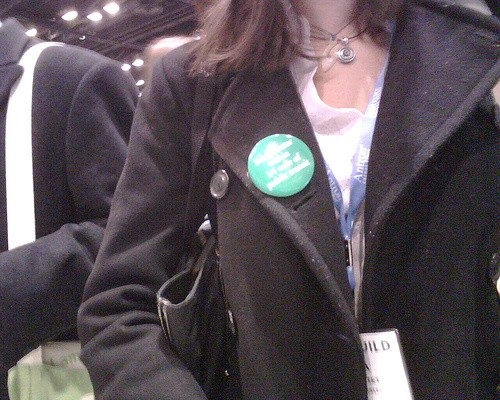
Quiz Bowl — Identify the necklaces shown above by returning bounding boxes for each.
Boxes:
[298,15,374,70]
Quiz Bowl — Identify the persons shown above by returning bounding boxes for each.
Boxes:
[0,18,139,400]
[76,0,500,400]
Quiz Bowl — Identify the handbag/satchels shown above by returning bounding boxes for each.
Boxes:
[150,235,240,400]
[6,339,96,399]
